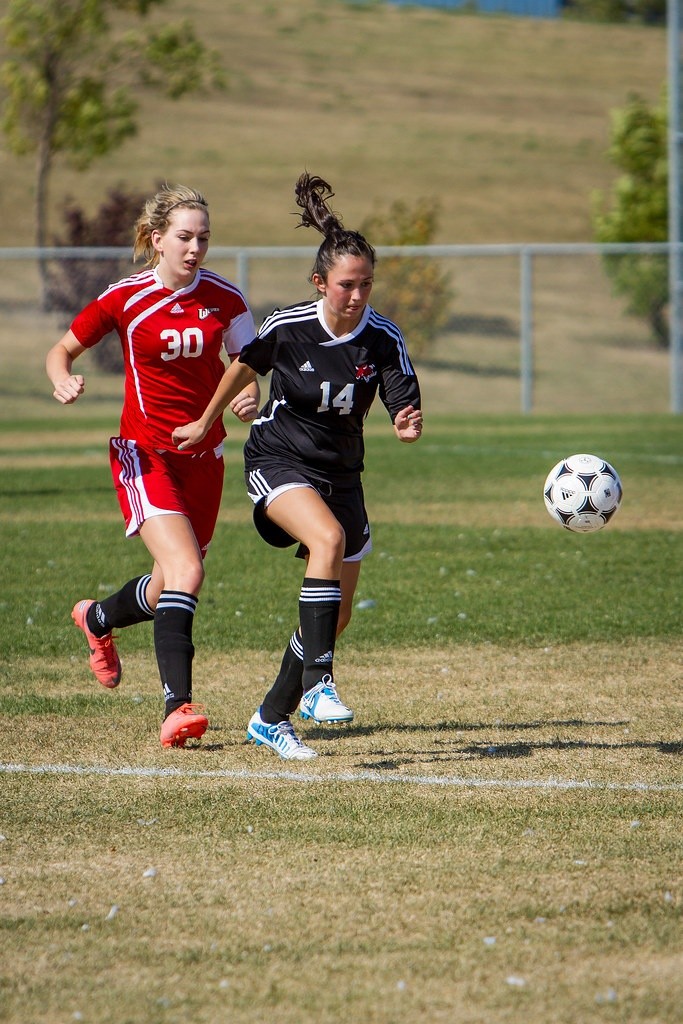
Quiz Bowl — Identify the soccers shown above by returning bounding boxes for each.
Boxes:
[543,454,623,535]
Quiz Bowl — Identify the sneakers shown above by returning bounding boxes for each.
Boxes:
[299,674,353,736]
[247,705,318,760]
[70,599,122,688]
[161,703,208,747]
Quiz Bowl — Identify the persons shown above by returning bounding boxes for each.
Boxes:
[171,173,423,760]
[46,184,259,749]
